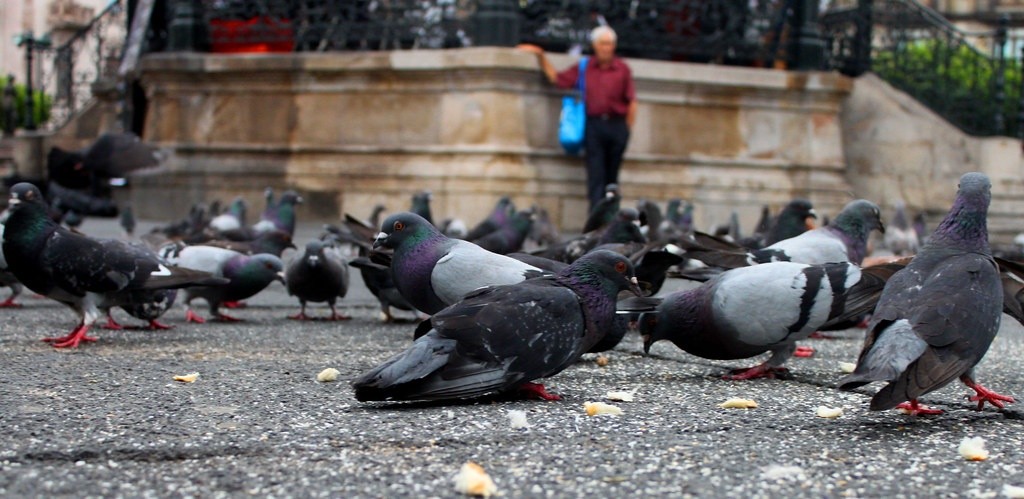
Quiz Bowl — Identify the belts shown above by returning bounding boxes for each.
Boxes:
[588,114,621,120]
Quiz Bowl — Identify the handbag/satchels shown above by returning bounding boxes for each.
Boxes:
[558,97,585,153]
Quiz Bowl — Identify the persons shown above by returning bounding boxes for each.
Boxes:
[515,26,636,213]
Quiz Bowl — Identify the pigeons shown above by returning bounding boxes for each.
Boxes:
[0,169,1015,420]
